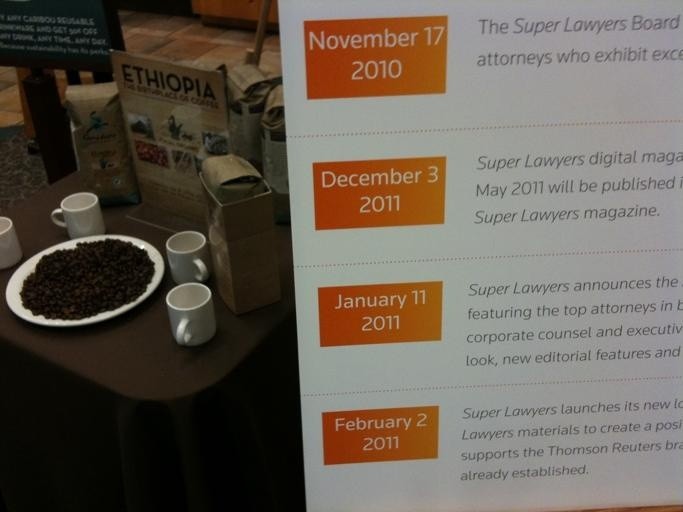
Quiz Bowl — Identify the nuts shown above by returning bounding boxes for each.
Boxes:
[19,238,155,320]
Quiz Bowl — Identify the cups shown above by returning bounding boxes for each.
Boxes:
[166,231,219,348]
[49,192,106,241]
[0,217,24,270]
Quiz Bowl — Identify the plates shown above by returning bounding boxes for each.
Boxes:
[5,235,166,329]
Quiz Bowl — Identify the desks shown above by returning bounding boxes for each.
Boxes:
[0,169,295,512]
